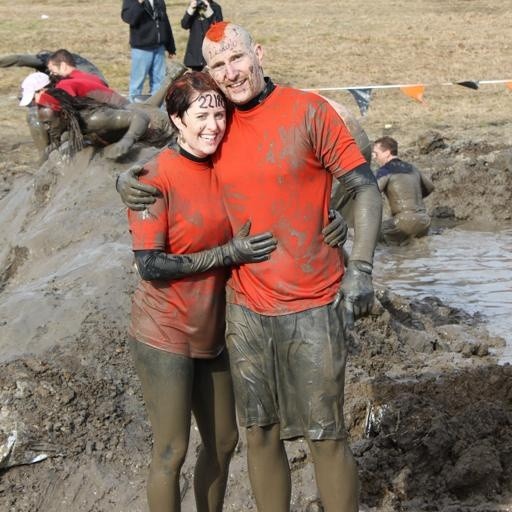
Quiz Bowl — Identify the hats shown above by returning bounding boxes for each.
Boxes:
[19,70,51,106]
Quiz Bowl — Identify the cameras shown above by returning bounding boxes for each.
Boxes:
[197,0,207,10]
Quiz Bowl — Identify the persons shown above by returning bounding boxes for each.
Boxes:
[116,22,381,511]
[121,0,177,111]
[371,136,433,243]
[126,69,349,510]
[0,48,188,161]
[182,0,223,70]
